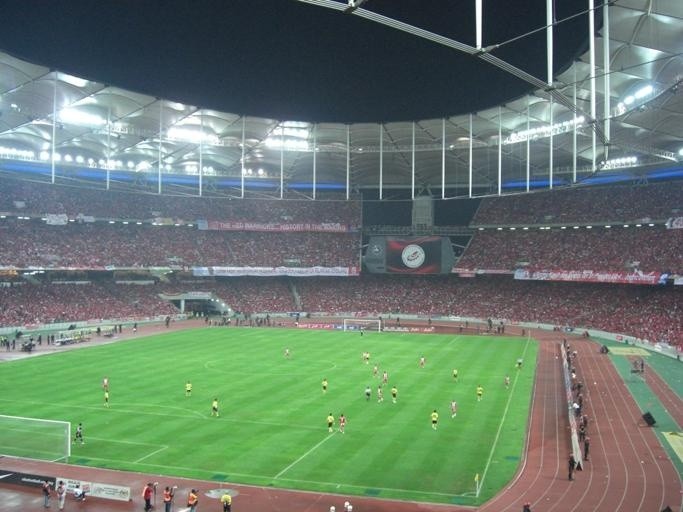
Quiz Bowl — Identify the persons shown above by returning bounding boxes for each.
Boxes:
[101,376,108,390]
[72,484,84,500]
[142,482,153,512]
[567,360,590,480]
[209,397,220,417]
[346,505,353,511]
[187,488,197,511]
[320,349,525,434]
[54,480,65,510]
[103,390,109,407]
[0,166,683,361]
[162,486,173,512]
[220,491,231,511]
[41,479,49,506]
[343,500,349,511]
[71,423,84,444]
[522,501,531,512]
[184,381,192,396]
[329,505,336,511]
